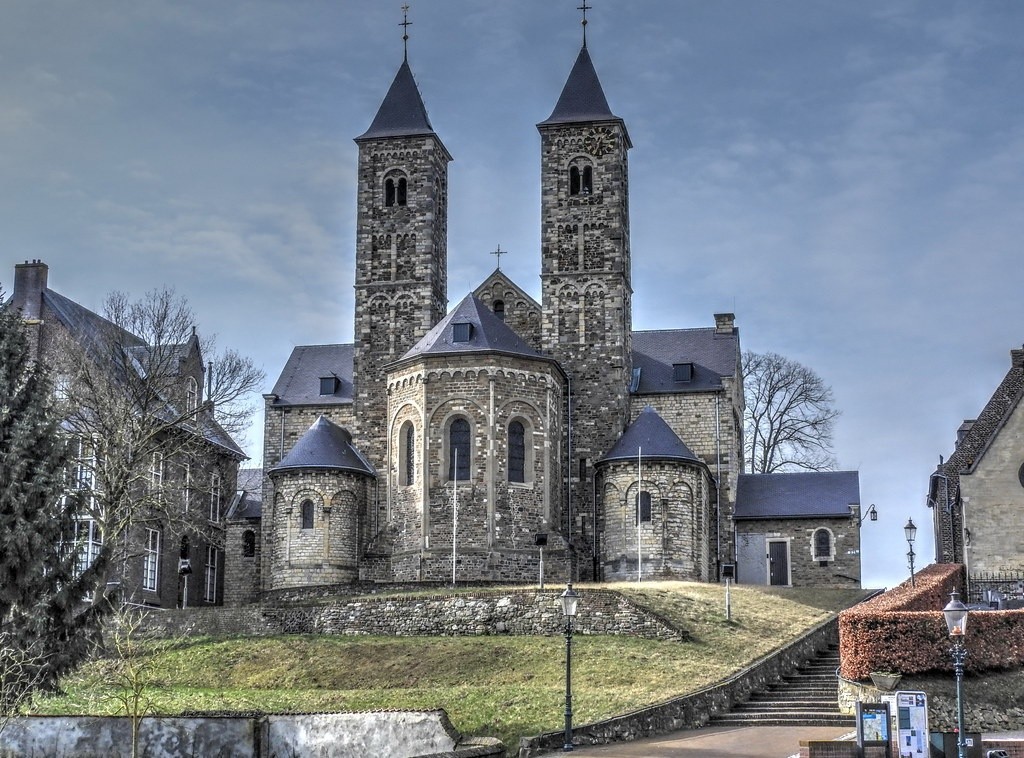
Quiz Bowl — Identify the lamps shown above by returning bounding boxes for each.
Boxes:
[179,563,194,610]
[721,564,734,622]
[860,504,877,527]
[533,533,549,588]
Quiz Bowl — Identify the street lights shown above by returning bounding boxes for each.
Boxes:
[904,517,917,585]
[559,584,580,751]
[943,586,970,758]
